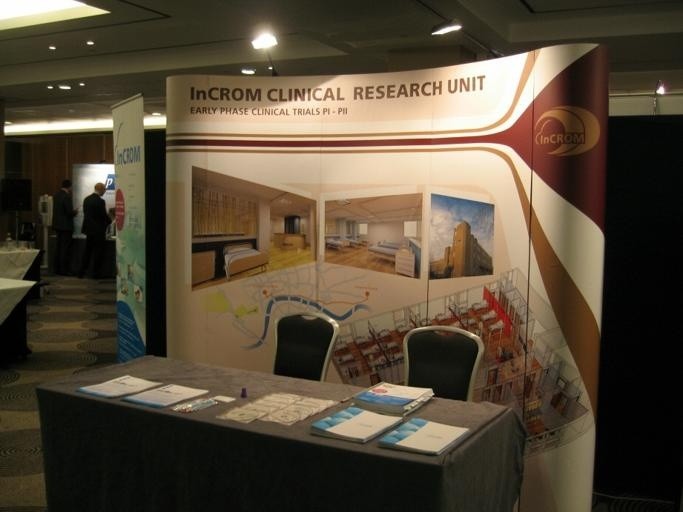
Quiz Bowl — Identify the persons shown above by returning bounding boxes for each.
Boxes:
[50,179,79,278]
[80,183,114,280]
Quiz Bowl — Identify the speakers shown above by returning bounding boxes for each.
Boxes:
[0,179,32,212]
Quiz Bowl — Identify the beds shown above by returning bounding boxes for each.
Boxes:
[218,240,272,283]
[323,230,409,264]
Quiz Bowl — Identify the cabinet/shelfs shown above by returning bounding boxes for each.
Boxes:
[0,274,49,369]
[0,243,47,302]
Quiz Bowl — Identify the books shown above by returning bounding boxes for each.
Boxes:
[309,405,404,444]
[74,374,163,400]
[120,384,209,409]
[350,380,434,417]
[375,418,471,455]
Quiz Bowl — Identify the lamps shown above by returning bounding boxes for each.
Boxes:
[427,16,464,38]
[248,27,286,78]
[650,79,666,115]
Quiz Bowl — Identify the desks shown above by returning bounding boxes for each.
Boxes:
[32,353,528,511]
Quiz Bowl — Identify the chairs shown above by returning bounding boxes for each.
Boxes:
[265,309,340,382]
[397,324,486,405]
